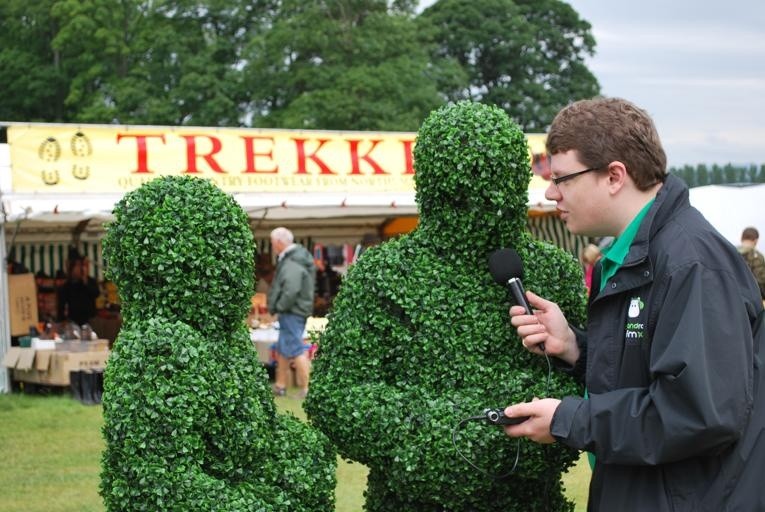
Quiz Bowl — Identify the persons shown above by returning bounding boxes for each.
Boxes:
[54,253,102,339]
[581,243,602,264]
[8,259,25,275]
[733,226,765,297]
[265,227,316,400]
[256,269,271,292]
[498,96,765,512]
[317,257,344,301]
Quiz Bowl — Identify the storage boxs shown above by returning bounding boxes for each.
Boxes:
[1,273,112,387]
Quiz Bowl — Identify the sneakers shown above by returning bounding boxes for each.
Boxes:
[273,386,287,396]
[291,389,308,399]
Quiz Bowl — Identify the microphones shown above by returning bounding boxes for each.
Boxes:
[488,248,545,351]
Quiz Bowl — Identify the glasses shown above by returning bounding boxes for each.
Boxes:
[549,166,606,189]
